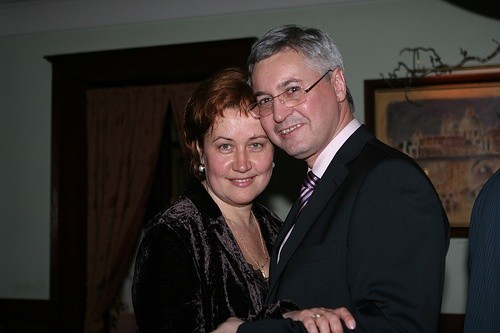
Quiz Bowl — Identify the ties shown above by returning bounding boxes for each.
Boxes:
[294,170,320,226]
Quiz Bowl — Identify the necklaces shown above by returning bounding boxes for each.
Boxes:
[222,207,269,270]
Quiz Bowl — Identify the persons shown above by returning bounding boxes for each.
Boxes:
[202,22,452,333]
[131,69,357,333]
[462,164,500,333]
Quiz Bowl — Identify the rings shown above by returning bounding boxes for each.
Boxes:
[311,313,324,322]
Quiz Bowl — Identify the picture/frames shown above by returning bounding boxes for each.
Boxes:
[364,72,500,240]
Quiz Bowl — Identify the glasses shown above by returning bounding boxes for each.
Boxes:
[248,69,334,118]
